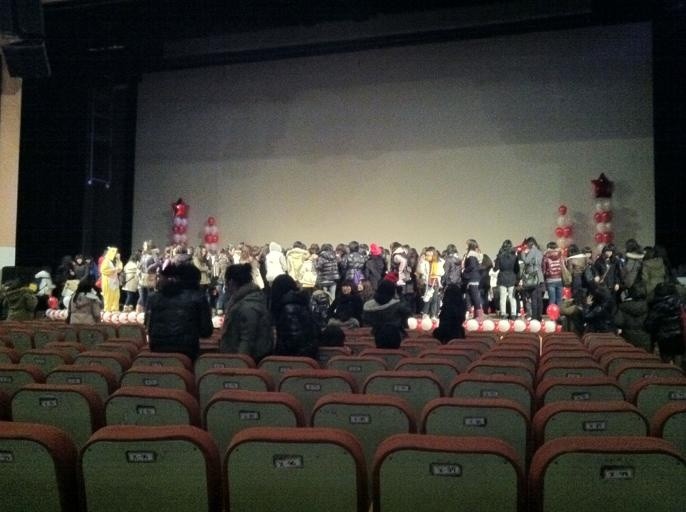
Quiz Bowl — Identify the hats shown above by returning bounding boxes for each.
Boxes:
[370,243,383,256]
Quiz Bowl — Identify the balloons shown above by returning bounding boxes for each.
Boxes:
[204,216,219,243]
[554,204,575,247]
[591,171,616,253]
[172,198,190,243]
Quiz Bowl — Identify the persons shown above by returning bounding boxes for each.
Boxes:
[3,239,686,368]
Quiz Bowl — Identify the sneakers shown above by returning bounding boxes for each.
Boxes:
[103,305,144,312]
[422,312,543,321]
